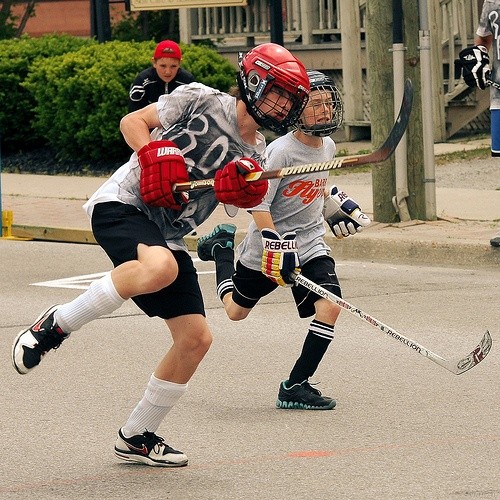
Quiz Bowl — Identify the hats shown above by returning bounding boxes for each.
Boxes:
[153,40,181,60]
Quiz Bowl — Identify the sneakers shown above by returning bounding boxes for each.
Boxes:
[113,427,188,467]
[11,304,70,374]
[275,380,336,410]
[196,224,237,261]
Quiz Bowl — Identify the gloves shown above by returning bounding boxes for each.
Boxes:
[323,185,372,239]
[459,45,494,90]
[260,228,303,287]
[214,158,268,208]
[137,139,190,211]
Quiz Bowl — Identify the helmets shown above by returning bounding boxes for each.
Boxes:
[293,70,342,137]
[237,42,310,136]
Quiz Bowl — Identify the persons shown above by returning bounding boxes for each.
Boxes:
[197,70,371,411]
[128,39,196,133]
[11,43,310,468]
[473,0,500,246]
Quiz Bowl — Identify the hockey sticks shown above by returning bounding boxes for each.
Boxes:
[485,78,500,92]
[171,77,413,193]
[296,272,493,376]
